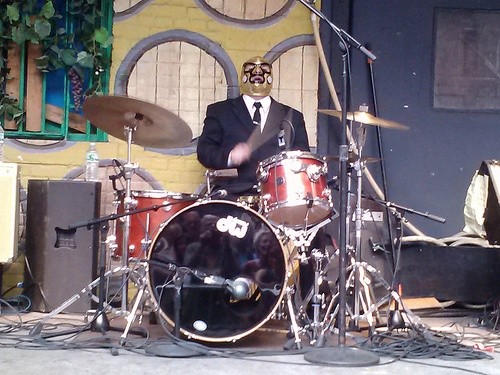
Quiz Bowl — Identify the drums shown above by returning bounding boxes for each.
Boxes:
[114,189,206,262]
[256,150,331,226]
[146,199,300,342]
[237,196,260,212]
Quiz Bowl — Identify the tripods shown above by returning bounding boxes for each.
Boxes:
[281,0,449,349]
[29,124,156,356]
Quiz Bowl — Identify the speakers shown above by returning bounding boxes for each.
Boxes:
[21,179,103,315]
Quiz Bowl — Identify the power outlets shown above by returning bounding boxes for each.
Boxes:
[362,212,384,222]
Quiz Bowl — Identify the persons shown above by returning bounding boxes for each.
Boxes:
[197,56,327,339]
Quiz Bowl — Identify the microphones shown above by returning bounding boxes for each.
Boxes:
[203,275,251,300]
[217,189,228,198]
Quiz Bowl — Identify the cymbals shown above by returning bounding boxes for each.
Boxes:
[314,109,410,131]
[329,153,380,162]
[82,96,194,149]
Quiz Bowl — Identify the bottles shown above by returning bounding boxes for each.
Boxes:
[85,143,99,181]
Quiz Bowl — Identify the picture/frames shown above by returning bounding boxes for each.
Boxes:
[429,6,500,114]
